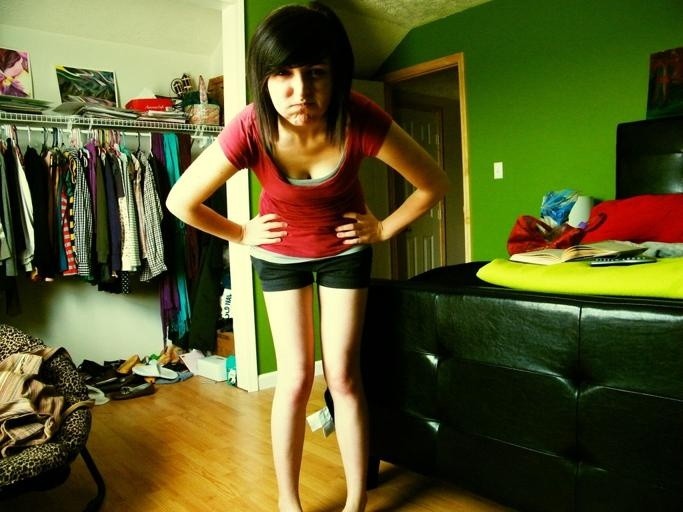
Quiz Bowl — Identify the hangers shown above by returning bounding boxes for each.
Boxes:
[0,117,146,156]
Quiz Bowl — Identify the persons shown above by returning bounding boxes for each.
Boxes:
[164,2,450,512]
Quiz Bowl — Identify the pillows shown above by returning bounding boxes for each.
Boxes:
[580,193,683,244]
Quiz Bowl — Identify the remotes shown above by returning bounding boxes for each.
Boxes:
[590,256,657,266]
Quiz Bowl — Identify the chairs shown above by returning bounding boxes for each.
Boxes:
[0,326,106,510]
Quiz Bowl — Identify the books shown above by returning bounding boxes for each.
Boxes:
[509,240,649,266]
[0,96,188,124]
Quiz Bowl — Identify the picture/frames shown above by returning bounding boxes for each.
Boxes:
[1,47,121,109]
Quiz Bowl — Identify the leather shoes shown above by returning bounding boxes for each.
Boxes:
[77,344,191,407]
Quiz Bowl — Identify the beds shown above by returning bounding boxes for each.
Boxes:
[358,118,683,510]
